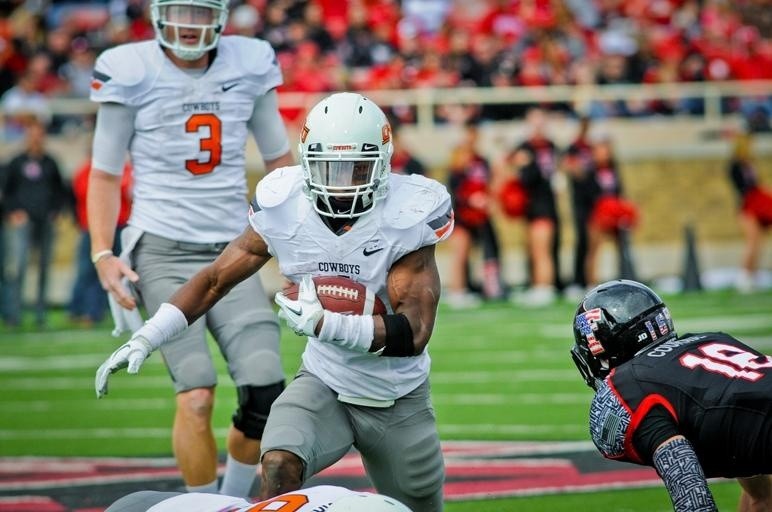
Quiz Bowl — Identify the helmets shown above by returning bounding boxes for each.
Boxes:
[568,278,676,394]
[299,91,396,219]
[150,1,231,63]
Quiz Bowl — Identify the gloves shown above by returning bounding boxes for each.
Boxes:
[274,274,326,339]
[94,333,154,399]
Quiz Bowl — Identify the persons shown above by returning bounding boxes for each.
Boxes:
[218,0,772,126]
[450,110,635,308]
[86,0,299,493]
[69,160,133,329]
[1,120,68,326]
[1,1,157,137]
[391,133,426,175]
[94,92,456,511]
[570,278,770,511]
[729,134,772,292]
[104,484,414,512]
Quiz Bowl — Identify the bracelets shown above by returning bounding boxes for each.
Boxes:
[372,309,414,358]
[317,309,375,351]
[90,249,114,262]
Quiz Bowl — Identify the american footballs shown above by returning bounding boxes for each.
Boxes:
[282,276,387,316]
